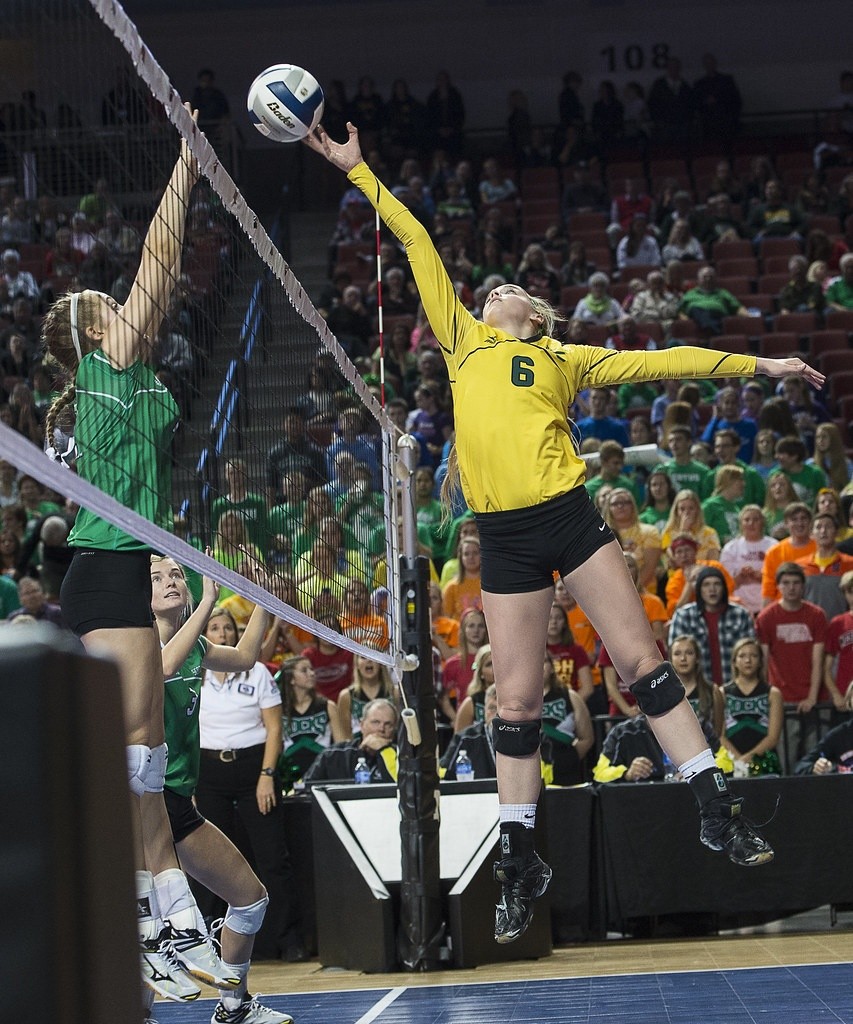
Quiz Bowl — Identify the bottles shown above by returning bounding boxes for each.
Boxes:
[354,757,370,784]
[455,750,473,781]
[663,751,677,782]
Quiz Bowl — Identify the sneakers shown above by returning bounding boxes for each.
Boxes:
[144,1007,160,1024]
[699,793,780,866]
[141,918,202,1003]
[494,849,552,944]
[210,992,295,1024]
[171,915,240,990]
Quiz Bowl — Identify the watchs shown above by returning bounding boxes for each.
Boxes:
[261,767,275,778]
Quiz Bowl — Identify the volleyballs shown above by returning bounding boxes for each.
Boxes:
[246,64,324,143]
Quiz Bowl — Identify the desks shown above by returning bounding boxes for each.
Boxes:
[213,774,853,963]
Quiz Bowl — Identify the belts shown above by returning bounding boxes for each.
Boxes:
[200,743,267,763]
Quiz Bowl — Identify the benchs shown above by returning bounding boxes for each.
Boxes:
[460,105,846,136]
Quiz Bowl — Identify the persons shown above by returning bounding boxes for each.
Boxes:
[303,117,829,942]
[37,101,298,1024]
[0,69,208,621]
[194,52,852,785]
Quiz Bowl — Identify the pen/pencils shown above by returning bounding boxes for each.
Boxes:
[820,751,824,757]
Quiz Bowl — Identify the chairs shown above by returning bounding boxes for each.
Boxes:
[18,218,224,304]
[338,156,853,456]
